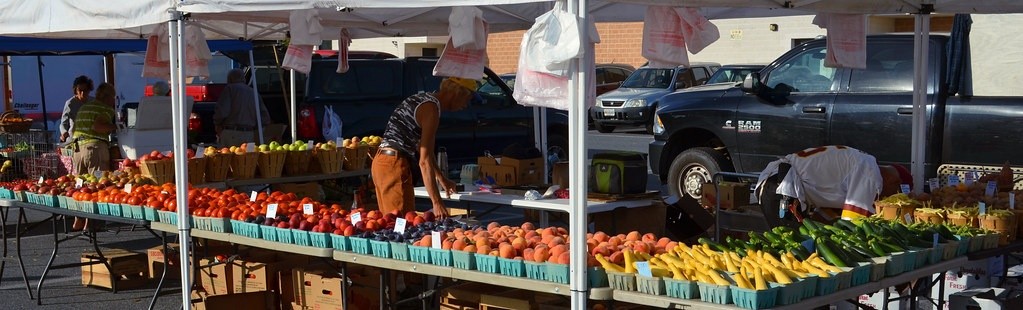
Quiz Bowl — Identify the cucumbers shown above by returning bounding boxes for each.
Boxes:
[696,216,959,266]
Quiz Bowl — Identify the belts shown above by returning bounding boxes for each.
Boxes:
[378,150,405,156]
[224,126,254,131]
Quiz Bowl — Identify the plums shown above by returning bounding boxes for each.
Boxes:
[246,206,435,236]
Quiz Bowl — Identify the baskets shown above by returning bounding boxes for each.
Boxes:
[1,143,1022,304]
[0,111,33,134]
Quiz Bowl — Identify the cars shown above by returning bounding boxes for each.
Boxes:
[477,63,637,99]
[703,63,830,93]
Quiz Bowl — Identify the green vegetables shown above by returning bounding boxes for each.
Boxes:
[12,141,30,151]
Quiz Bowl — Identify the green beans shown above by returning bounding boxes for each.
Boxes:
[876,190,1016,219]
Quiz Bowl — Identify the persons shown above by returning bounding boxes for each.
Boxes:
[59,75,94,141]
[214,69,270,147]
[371,77,477,222]
[755,144,899,232]
[71,82,116,229]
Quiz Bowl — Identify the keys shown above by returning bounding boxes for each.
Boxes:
[779,198,790,212]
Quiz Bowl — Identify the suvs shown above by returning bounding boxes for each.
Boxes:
[589,61,728,134]
[237,51,568,188]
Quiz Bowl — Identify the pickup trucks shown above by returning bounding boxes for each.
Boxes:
[646,32,1022,218]
[143,51,289,145]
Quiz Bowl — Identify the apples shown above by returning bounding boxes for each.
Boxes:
[13,168,152,197]
[123,135,383,166]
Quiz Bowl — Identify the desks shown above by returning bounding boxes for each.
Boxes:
[0,167,1023,310]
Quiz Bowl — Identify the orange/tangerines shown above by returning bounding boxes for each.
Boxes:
[72,182,341,221]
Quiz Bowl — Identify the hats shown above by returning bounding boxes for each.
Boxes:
[891,163,913,192]
[449,77,477,92]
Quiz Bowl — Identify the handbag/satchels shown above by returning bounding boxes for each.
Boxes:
[321,106,342,141]
[590,150,648,196]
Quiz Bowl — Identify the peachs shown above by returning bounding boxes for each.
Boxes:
[413,222,678,267]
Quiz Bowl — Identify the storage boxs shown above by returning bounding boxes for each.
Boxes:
[440,282,571,310]
[552,159,593,189]
[276,262,380,310]
[187,243,307,310]
[477,153,544,186]
[701,182,750,208]
[148,243,181,279]
[931,255,1004,298]
[115,95,195,160]
[145,82,228,101]
[81,250,148,292]
[590,150,650,197]
